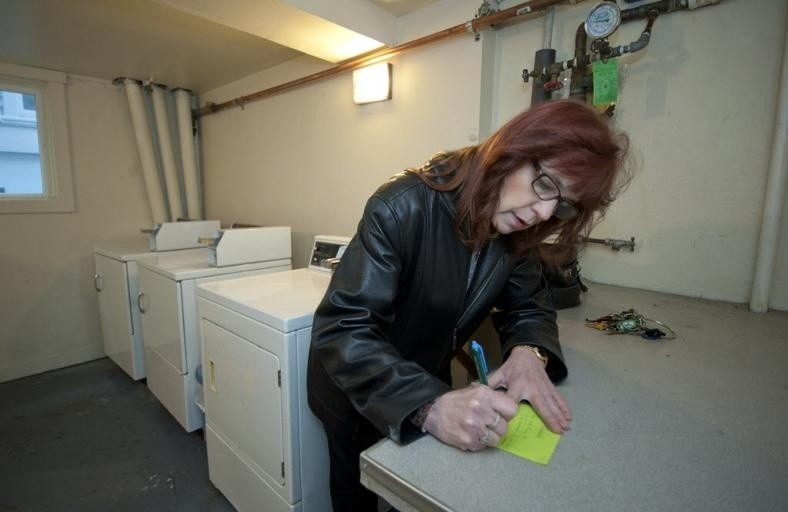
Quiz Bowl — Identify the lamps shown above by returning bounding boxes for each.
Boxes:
[351,62,391,104]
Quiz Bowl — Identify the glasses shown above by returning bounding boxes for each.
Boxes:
[531,158,580,221]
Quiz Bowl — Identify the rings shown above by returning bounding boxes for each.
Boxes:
[478,428,489,447]
[487,410,501,430]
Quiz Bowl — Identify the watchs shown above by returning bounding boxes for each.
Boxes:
[513,345,548,366]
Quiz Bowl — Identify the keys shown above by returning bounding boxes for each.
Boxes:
[583,308,647,336]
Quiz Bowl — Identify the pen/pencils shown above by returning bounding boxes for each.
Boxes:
[471,341,488,386]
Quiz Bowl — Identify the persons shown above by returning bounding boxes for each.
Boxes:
[306,95,641,512]
